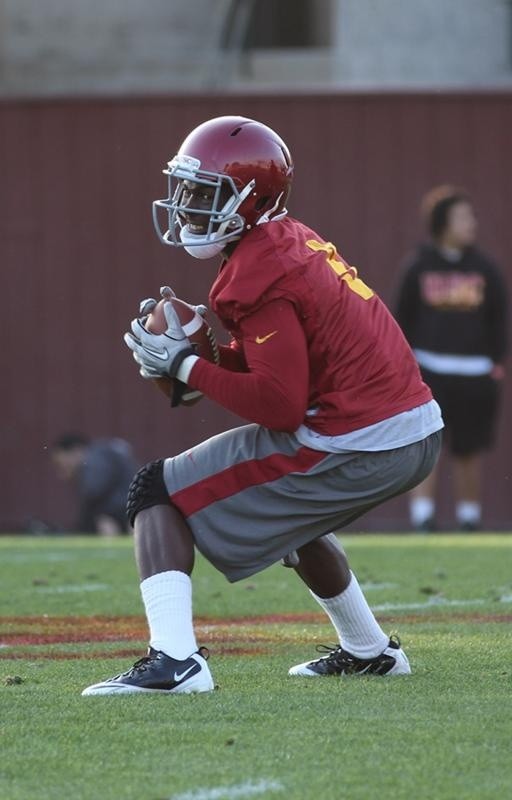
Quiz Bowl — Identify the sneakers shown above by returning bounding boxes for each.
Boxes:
[287,635,412,676]
[81,646,215,696]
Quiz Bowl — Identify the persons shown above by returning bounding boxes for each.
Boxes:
[22,418,164,538]
[389,194,510,534]
[75,111,450,699]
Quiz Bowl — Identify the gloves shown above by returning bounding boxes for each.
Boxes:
[123,285,208,382]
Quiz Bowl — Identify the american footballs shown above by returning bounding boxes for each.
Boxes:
[145,295,220,407]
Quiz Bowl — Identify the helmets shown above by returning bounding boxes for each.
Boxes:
[171,115,294,234]
